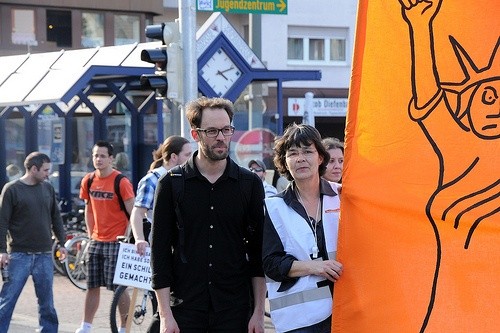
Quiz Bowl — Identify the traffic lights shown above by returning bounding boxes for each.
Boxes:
[139,22,183,103]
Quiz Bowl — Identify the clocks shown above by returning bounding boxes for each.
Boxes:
[198,47,243,98]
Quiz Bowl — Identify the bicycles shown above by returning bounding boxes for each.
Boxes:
[52,226,157,333]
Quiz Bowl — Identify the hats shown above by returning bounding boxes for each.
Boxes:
[248,160,266,172]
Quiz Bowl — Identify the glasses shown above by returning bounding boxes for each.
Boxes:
[249,167,263,172]
[195,126,235,138]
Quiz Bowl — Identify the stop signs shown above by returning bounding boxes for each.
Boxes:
[234,128,276,173]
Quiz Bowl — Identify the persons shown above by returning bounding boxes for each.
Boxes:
[147,97,267,333]
[130,136,192,314]
[75,141,135,333]
[248,158,279,198]
[6,163,22,181]
[260,121,343,333]
[0,151,68,333]
[319,137,345,184]
